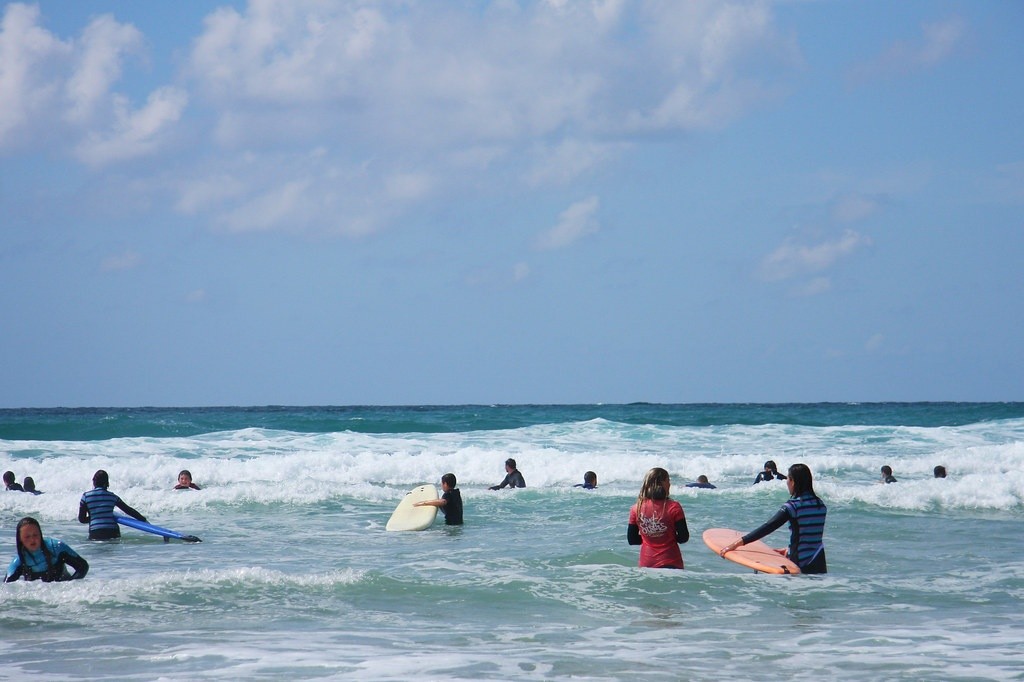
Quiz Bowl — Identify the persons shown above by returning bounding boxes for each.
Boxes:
[627,468,689,569]
[413,473,463,525]
[488,459,526,490]
[78,470,150,541]
[3,471,42,495]
[174,470,201,491]
[686,475,717,489]
[4,517,89,582]
[574,471,599,490]
[720,460,828,574]
[881,466,897,483]
[934,466,946,478]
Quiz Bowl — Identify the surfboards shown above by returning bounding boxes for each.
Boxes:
[385,485,440,531]
[114,511,201,542]
[701,527,801,575]
